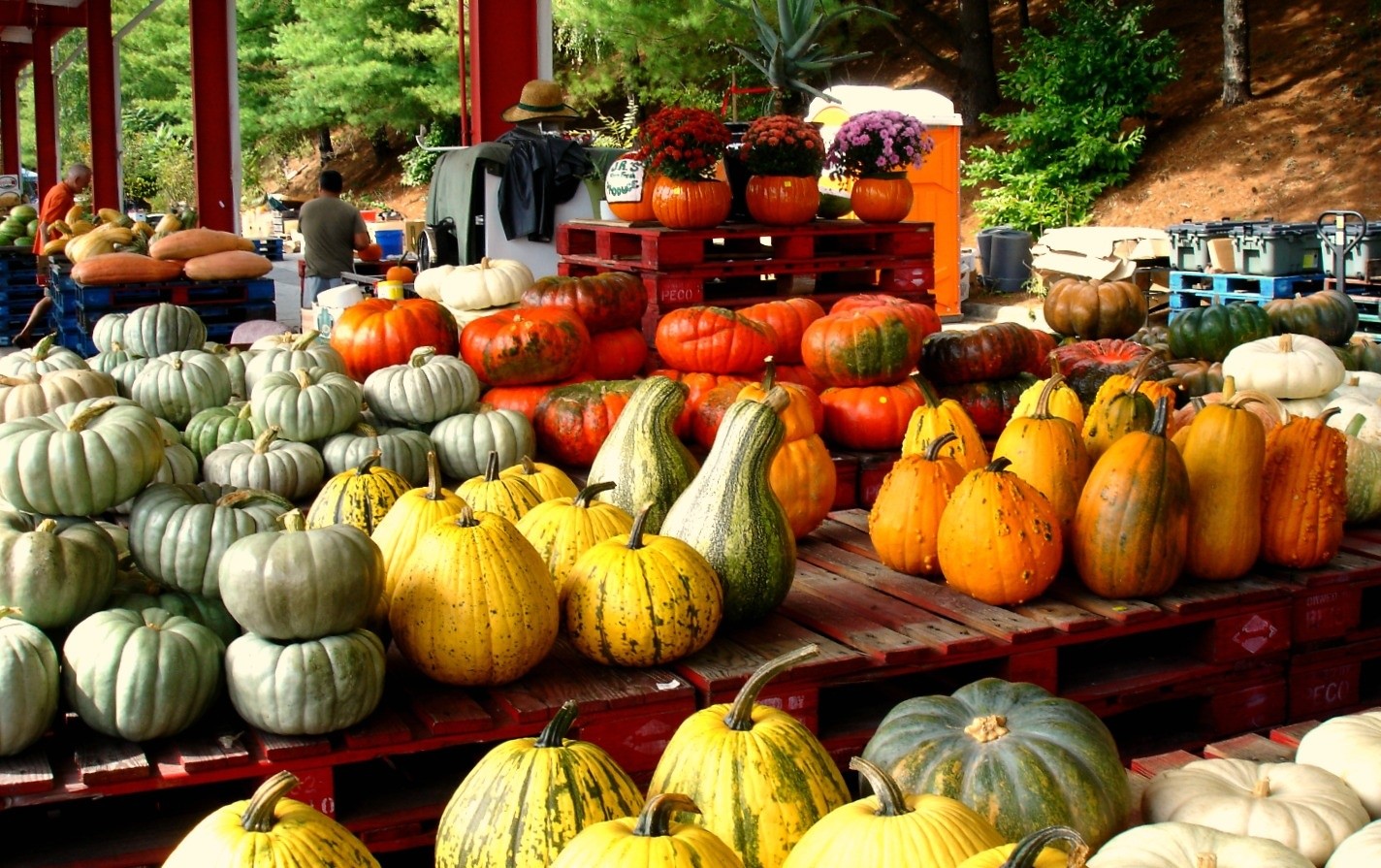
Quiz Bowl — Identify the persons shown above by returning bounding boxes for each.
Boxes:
[297,171,369,308]
[13,164,91,347]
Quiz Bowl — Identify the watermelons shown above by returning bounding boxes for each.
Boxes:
[0,205,40,247]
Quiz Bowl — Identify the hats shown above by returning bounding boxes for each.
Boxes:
[501,77,582,122]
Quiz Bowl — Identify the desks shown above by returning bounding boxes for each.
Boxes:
[551,218,936,334]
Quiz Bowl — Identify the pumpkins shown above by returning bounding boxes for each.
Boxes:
[604,150,670,222]
[824,280,1380,605]
[42,205,274,286]
[652,175,914,230]
[160,644,1381,868]
[0,258,943,764]
[358,242,383,261]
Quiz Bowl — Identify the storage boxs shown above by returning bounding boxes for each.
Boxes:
[960,262,969,302]
[961,246,976,283]
[0,256,276,360]
[1166,215,1274,272]
[1227,221,1335,275]
[1316,220,1381,278]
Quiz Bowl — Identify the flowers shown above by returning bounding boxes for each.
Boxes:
[633,107,732,184]
[740,111,828,182]
[823,109,935,191]
